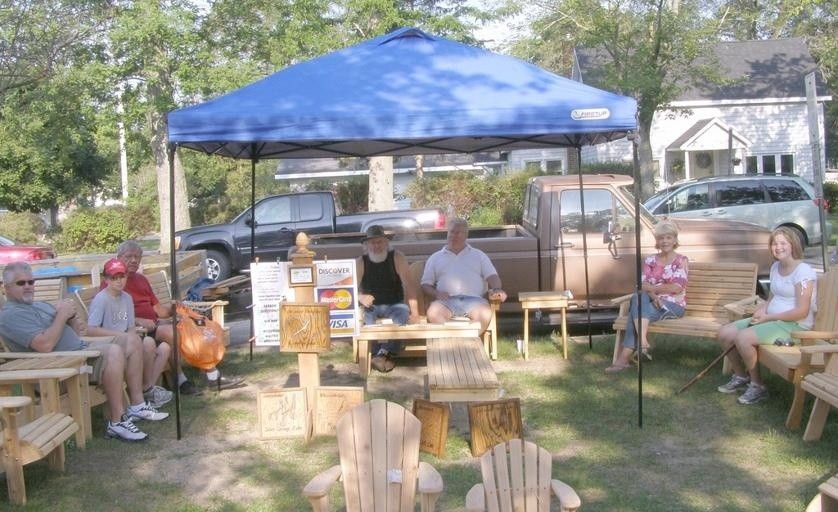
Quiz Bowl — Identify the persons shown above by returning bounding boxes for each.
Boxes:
[99,241,220,396]
[604,220,689,373]
[354,225,419,373]
[86,259,174,409]
[717,227,817,405]
[420,219,508,337]
[0,262,169,440]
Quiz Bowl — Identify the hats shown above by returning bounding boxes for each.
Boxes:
[360,226,395,243]
[103,259,127,276]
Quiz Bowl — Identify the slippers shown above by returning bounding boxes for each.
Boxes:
[633,347,652,363]
[604,363,633,374]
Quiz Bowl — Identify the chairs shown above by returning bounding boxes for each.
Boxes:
[803,471,838,511]
[0,296,134,438]
[0,367,79,507]
[465,439,582,512]
[302,399,443,512]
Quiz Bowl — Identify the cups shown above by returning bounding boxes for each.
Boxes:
[517,340,524,354]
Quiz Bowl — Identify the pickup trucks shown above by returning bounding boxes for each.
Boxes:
[175,191,446,302]
[287,173,777,314]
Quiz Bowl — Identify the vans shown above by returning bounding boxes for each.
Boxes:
[643,176,828,249]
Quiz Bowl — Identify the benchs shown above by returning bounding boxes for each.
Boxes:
[74,268,232,392]
[756,264,837,442]
[610,262,762,376]
[350,261,501,360]
[0,276,69,302]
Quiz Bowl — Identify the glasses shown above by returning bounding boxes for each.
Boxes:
[5,280,34,285]
[776,338,795,347]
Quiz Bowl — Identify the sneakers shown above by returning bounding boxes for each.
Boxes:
[738,381,770,404]
[104,385,173,441]
[718,374,750,393]
[371,353,400,372]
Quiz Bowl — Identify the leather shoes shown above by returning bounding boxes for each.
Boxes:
[180,380,202,395]
[207,377,245,391]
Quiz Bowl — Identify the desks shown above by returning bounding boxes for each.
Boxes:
[519,291,568,361]
[426,337,500,403]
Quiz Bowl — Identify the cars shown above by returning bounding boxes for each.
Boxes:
[1,235,56,295]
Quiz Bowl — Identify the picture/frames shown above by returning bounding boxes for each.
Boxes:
[467,398,524,458]
[412,398,449,457]
[310,384,364,436]
[287,264,317,287]
[278,302,330,353]
[256,387,307,440]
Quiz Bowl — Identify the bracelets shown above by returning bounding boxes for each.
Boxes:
[493,288,501,291]
